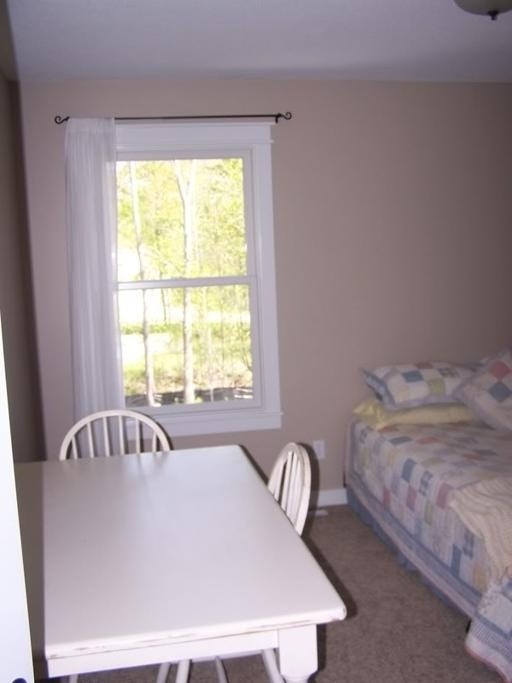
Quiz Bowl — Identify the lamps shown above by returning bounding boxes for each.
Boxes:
[455,0,512,21]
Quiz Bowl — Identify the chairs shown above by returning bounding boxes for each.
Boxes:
[57,408,172,683]
[157,442,314,683]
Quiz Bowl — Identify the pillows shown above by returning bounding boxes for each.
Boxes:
[455,346,512,432]
[355,396,476,430]
[362,357,474,412]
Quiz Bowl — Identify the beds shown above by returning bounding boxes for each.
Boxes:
[346,417,512,683]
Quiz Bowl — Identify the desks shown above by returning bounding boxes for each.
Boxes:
[16,444,348,682]
